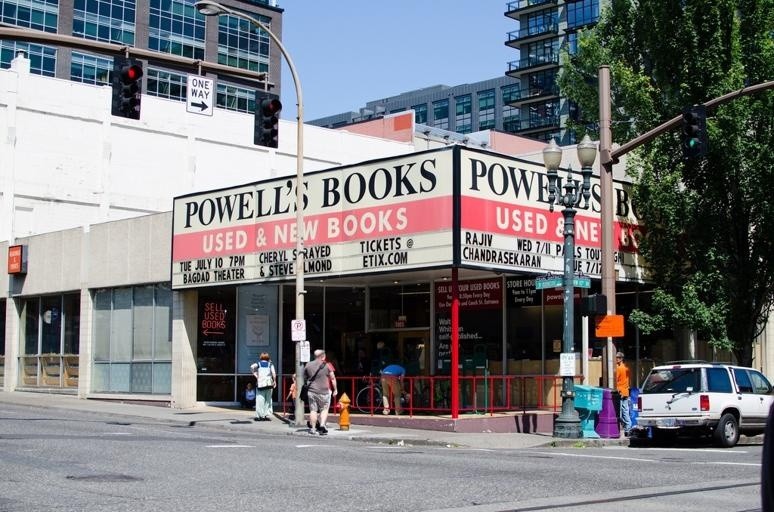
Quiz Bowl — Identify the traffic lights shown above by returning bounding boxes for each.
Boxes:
[252,89,282,149]
[681,104,709,159]
[111,54,145,121]
[583,294,609,317]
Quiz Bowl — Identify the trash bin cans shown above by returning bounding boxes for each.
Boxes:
[628,386,639,425]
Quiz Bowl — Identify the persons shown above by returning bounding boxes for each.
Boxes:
[286,373,298,421]
[250,351,278,422]
[304,349,330,436]
[382,365,407,416]
[615,351,635,434]
[245,382,256,410]
[307,351,339,428]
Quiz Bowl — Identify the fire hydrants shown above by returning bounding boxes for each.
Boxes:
[336,392,352,430]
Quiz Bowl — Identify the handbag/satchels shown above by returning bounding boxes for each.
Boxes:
[300,383,309,401]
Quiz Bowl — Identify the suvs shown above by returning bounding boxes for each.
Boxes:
[633,359,774,449]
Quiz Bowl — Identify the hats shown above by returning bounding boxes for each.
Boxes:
[616,352,625,358]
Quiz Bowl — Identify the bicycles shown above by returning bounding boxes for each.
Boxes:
[356,379,416,415]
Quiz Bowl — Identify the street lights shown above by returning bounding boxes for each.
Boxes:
[542,130,597,438]
[190,1,308,432]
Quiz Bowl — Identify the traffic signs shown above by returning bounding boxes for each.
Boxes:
[573,277,592,289]
[185,74,216,117]
[536,278,564,291]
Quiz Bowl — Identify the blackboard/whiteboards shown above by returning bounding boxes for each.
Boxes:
[506,276,544,306]
[432,307,503,370]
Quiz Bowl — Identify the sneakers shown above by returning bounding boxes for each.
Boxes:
[260,416,271,421]
[309,424,328,434]
